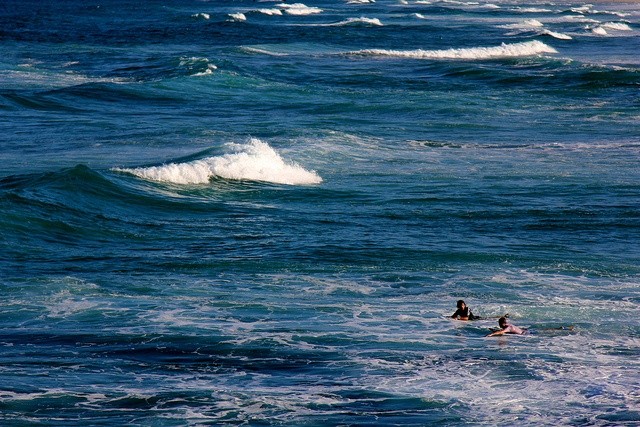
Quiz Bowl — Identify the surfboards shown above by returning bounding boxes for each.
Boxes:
[488,327,538,338]
[439,314,511,323]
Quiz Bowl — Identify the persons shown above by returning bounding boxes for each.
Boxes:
[486,314,538,338]
[452,300,482,321]
